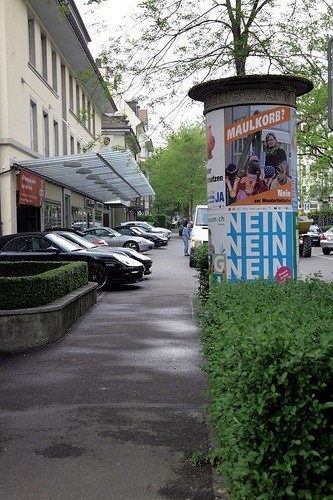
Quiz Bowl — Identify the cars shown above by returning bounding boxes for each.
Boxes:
[1,220,173,292]
[298,222,333,258]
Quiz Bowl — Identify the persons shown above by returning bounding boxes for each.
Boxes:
[225,156,291,205]
[264,133,287,180]
[182,223,192,256]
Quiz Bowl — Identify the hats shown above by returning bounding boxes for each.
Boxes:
[226,163,238,177]
[264,165,275,178]
[249,155,259,162]
[249,164,261,177]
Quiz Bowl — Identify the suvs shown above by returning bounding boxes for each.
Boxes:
[188,204,210,268]
